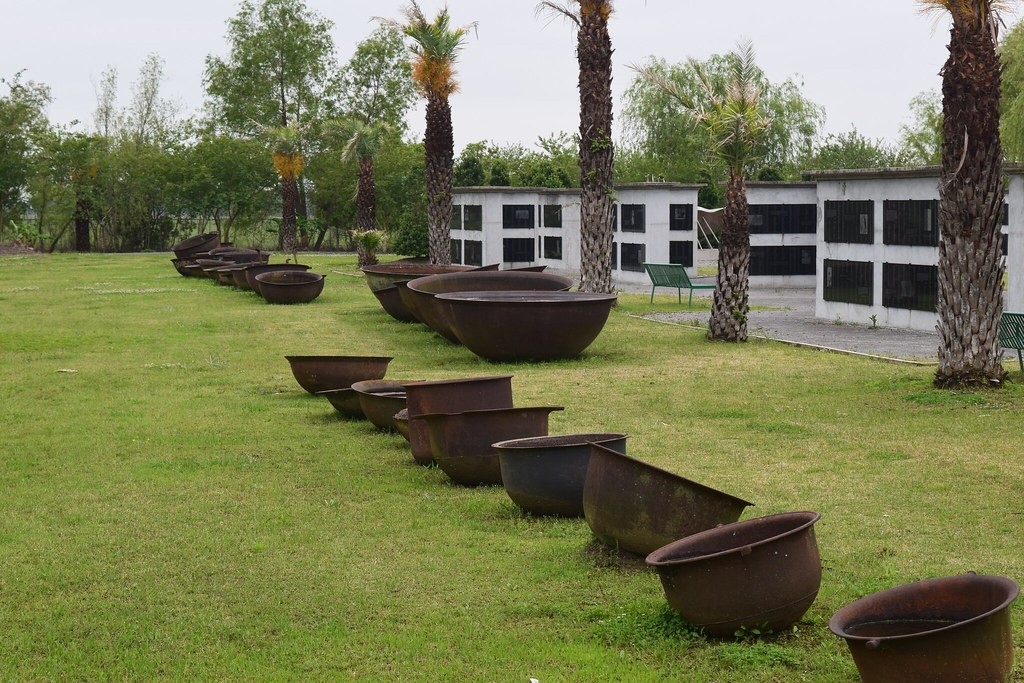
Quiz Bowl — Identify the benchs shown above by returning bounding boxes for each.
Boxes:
[643,261,715,305]
[999,313,1024,371]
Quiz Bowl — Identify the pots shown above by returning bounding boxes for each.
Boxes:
[316,388,367,419]
[491,433,631,517]
[351,379,427,430]
[435,290,618,363]
[284,356,394,397]
[360,263,483,300]
[646,511,822,640]
[401,374,514,467]
[170,231,327,305]
[583,440,756,557]
[407,271,574,346]
[393,265,548,329]
[374,263,500,323]
[393,408,411,442]
[830,571,1020,683]
[411,406,564,486]
